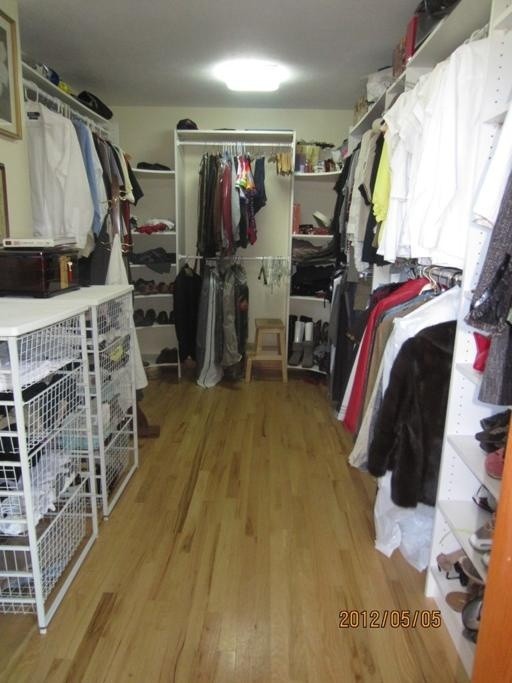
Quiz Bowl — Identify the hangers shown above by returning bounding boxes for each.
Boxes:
[23,81,109,142]
[470,27,482,41]
[259,256,287,293]
[185,253,244,268]
[461,35,470,47]
[200,140,292,158]
[399,264,462,297]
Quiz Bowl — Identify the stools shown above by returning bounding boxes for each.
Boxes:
[254,318,286,355]
[245,344,288,385]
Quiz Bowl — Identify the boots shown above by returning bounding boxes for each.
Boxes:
[288,315,330,371]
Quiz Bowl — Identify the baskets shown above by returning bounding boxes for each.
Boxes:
[1,294,131,616]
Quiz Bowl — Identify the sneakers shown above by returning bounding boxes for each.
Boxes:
[156,347,177,363]
[134,309,175,326]
[129,279,175,295]
[468,512,496,554]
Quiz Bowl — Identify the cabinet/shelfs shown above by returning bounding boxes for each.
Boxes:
[0,285,138,637]
[123,168,179,381]
[174,129,296,381]
[289,171,344,376]
[347,0,512,682]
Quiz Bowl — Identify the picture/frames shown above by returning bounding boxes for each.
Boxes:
[0,163,10,244]
[0,9,23,140]
